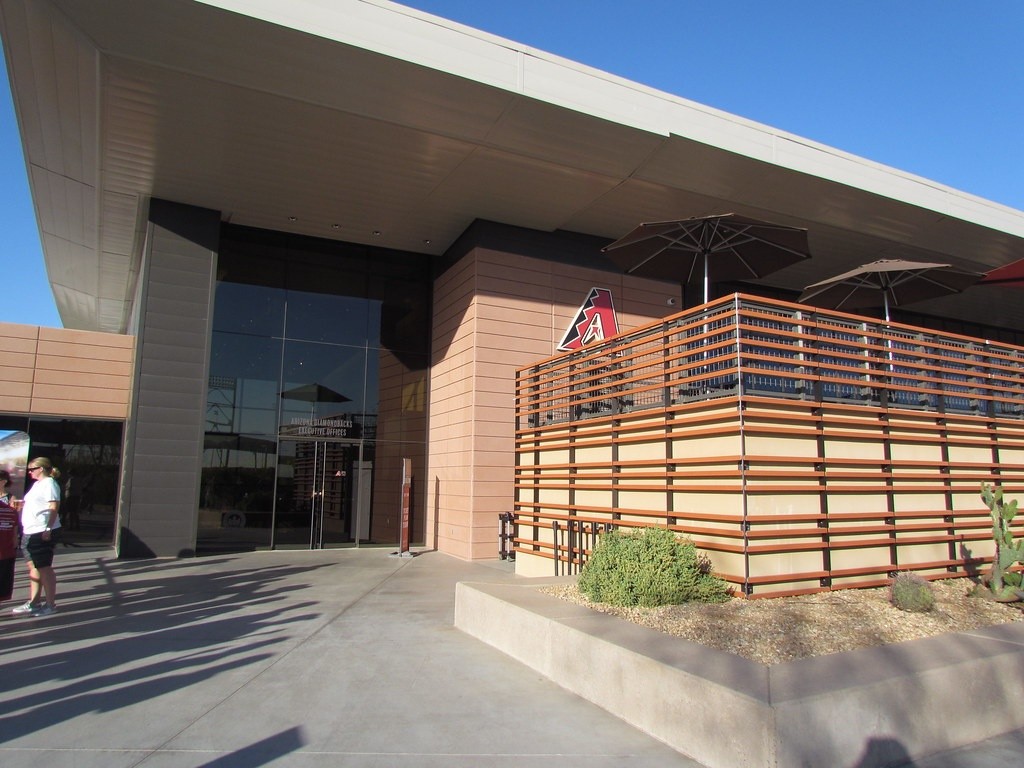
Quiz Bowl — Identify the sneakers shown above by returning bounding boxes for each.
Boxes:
[30,602,58,616]
[13,600,43,613]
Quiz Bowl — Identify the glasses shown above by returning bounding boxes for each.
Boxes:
[27,467,41,473]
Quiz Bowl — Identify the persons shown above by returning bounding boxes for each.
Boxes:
[0,470,18,509]
[13,457,62,617]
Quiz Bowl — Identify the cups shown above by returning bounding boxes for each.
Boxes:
[13,500,22,508]
